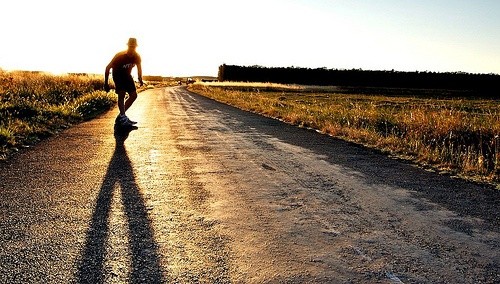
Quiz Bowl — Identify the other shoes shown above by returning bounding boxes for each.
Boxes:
[115,114,131,128]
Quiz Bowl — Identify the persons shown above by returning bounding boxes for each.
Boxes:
[104,38,143,125]
